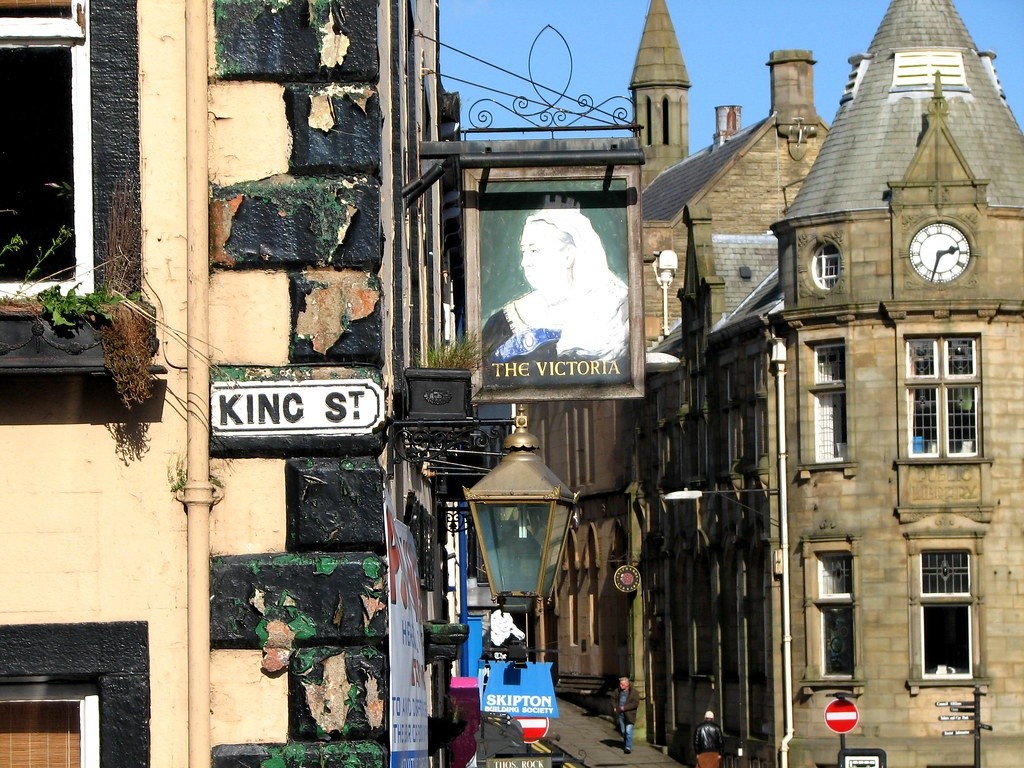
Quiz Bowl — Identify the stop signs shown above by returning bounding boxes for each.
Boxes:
[825,700,857,735]
[511,715,549,745]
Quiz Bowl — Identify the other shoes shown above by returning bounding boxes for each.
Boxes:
[621,740,633,753]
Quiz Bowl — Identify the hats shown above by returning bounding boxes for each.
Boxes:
[705,711,714,720]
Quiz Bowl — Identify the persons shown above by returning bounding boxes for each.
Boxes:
[611,677,640,754]
[695,711,724,768]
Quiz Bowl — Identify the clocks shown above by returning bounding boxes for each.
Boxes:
[911,223,971,284]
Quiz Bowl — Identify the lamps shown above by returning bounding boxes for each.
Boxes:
[386,405,580,610]
[664,488,781,524]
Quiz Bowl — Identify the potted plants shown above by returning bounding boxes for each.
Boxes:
[0,175,242,410]
